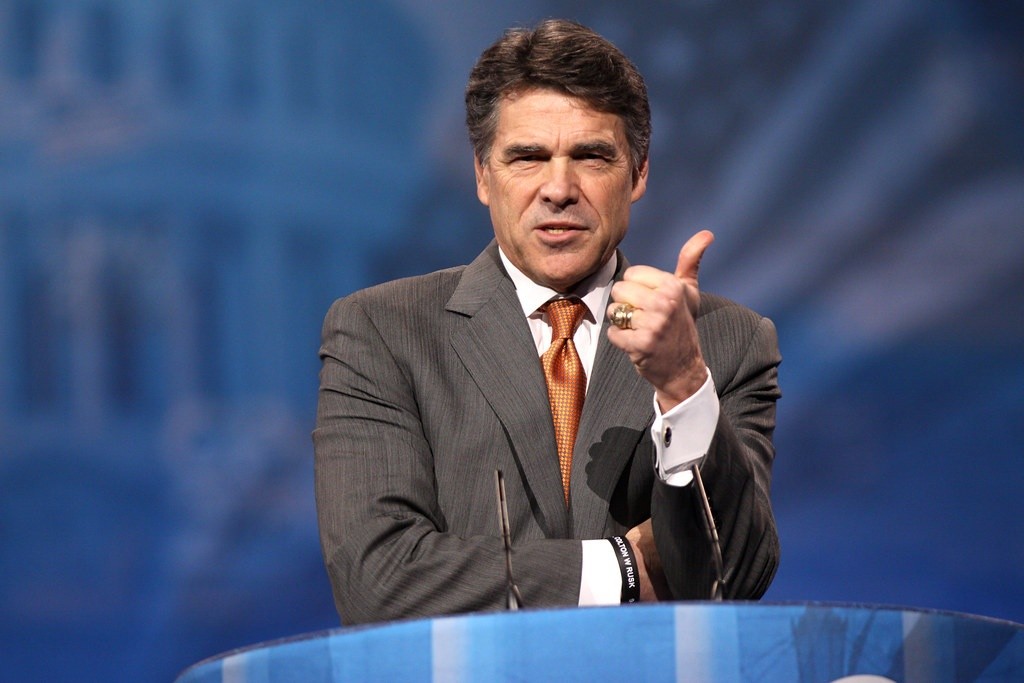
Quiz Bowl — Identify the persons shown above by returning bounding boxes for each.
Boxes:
[315,17,783,627]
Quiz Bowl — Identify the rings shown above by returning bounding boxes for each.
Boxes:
[611,300,638,331]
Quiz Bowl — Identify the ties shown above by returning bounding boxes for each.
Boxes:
[539,299,588,510]
